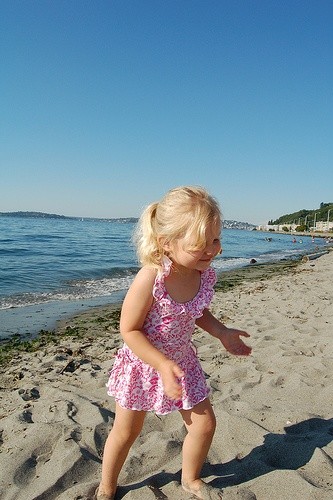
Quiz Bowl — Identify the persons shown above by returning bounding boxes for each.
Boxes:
[95,188,252,500]
